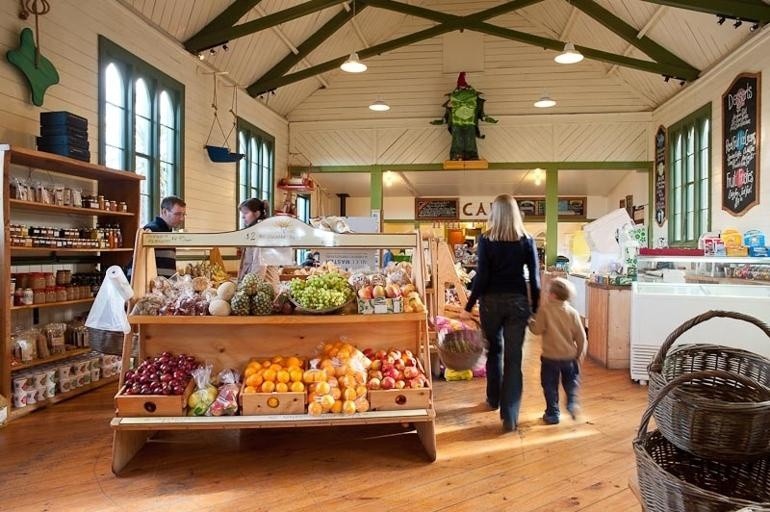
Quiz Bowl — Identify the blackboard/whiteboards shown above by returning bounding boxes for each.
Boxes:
[415,198,459,220]
[654,125,668,227]
[721,72,761,217]
[513,197,587,219]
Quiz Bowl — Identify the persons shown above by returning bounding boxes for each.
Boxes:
[234,198,282,295]
[457,192,543,432]
[523,275,590,425]
[299,250,321,267]
[383,248,394,267]
[124,195,189,300]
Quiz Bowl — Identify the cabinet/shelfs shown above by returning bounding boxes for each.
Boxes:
[110,216,436,474]
[429,234,479,322]
[0,143,146,424]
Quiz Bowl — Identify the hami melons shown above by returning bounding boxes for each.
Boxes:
[192,277,235,316]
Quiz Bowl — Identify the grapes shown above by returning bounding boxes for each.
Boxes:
[289,272,352,310]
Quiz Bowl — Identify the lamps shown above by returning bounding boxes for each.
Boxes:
[369,98,391,112]
[197,40,229,60]
[716,13,760,32]
[662,74,686,87]
[341,0,367,74]
[533,95,556,110]
[555,38,583,65]
[531,166,543,185]
[257,88,278,99]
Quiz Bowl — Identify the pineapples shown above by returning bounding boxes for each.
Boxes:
[230,271,275,315]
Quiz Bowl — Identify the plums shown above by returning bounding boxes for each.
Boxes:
[125,351,205,411]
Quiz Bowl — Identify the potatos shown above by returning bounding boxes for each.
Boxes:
[130,277,172,316]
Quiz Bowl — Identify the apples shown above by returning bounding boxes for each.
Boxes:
[363,346,430,405]
[358,283,425,313]
[189,368,241,415]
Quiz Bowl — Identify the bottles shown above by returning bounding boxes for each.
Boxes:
[98,195,105,210]
[64,321,89,349]
[17,269,91,306]
[99,223,123,247]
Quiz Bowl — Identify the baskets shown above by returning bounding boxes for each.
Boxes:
[436,315,486,369]
[648,311,770,461]
[88,329,139,359]
[287,281,356,315]
[631,370,770,511]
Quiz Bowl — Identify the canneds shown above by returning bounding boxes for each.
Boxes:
[10,269,93,307]
[81,194,128,212]
[9,223,111,248]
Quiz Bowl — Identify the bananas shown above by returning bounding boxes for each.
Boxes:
[208,261,232,288]
